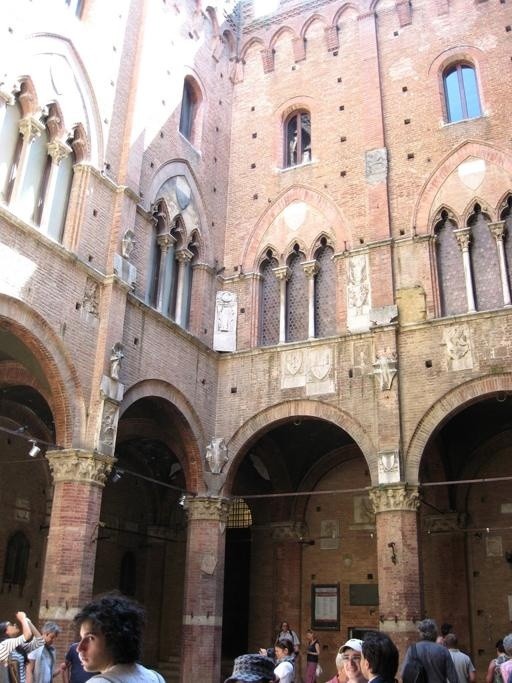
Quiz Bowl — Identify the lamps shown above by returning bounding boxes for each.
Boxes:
[112,470,122,483]
[179,496,186,507]
[28,440,41,458]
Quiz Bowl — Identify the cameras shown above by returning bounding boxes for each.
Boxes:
[257,647,276,657]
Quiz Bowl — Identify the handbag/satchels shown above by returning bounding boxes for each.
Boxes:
[316,665,322,676]
[403,659,428,683]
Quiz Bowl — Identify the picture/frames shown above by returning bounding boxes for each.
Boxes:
[311,583,341,632]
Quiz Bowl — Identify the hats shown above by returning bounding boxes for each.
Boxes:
[339,639,363,653]
[224,655,276,682]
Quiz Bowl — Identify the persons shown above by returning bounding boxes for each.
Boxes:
[0,612,46,683]
[73,590,166,683]
[65,642,101,683]
[224,619,512,683]
[26,621,71,683]
